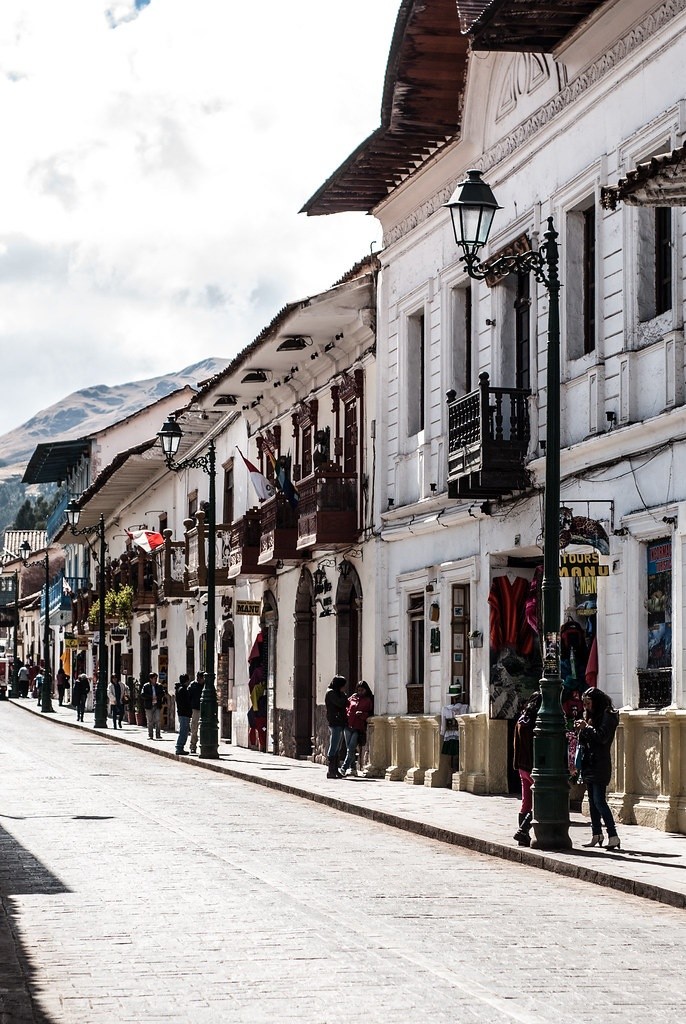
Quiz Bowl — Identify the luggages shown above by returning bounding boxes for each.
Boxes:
[81,719,84,722]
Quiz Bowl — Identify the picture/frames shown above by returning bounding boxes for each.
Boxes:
[454,653,463,662]
[454,607,463,616]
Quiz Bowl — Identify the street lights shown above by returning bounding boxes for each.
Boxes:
[0,562,21,697]
[19,537,55,714]
[444,167,577,851]
[160,411,222,762]
[65,497,110,729]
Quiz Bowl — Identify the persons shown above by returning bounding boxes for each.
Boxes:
[340,681,374,777]
[186,671,205,753]
[514,691,542,846]
[325,675,351,779]
[174,674,192,756]
[574,687,621,850]
[10,662,164,741]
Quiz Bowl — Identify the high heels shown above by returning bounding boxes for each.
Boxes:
[582,834,604,847]
[604,836,620,851]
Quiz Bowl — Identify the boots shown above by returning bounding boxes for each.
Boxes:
[514,812,533,847]
[327,755,342,779]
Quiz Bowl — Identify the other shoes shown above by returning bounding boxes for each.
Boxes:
[339,768,346,776]
[147,737,153,740]
[114,725,117,729]
[59,702,63,706]
[23,696,29,698]
[191,749,196,753]
[347,767,358,777]
[176,751,189,755]
[77,717,79,721]
[156,737,162,739]
[37,704,42,706]
[118,722,122,728]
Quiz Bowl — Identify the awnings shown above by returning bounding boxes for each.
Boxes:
[20,441,91,484]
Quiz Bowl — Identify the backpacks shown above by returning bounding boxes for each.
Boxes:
[561,616,590,662]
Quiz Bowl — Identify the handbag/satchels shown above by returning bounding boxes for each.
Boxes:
[123,684,131,705]
[574,745,585,770]
[64,674,70,689]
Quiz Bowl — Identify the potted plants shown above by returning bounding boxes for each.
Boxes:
[128,703,146,727]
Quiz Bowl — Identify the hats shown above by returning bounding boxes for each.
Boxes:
[446,685,466,696]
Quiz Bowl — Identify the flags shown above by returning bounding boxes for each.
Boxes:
[60,573,72,597]
[238,452,275,504]
[126,529,163,554]
[260,440,299,517]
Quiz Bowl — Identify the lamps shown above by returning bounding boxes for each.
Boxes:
[280,455,291,470]
[605,411,616,422]
[91,551,98,561]
[320,610,336,617]
[537,440,546,449]
[425,579,437,592]
[430,483,437,491]
[340,547,364,580]
[486,319,496,326]
[200,333,344,419]
[521,298,531,306]
[314,558,337,583]
[315,430,328,445]
[388,498,394,506]
